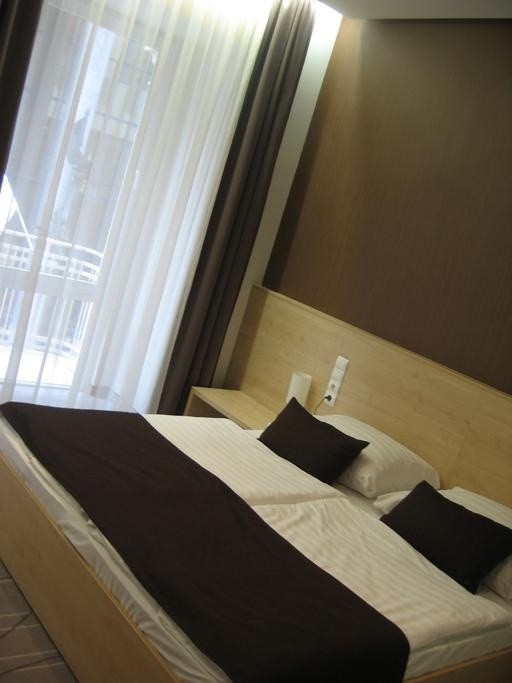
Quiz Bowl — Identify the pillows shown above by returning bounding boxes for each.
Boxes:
[309,414,441,500]
[372,485,512,603]
[377,480,512,594]
[256,396,370,484]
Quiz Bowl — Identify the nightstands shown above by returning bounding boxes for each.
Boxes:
[183,386,281,429]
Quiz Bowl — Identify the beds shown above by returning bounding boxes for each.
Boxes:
[0,385,512,683]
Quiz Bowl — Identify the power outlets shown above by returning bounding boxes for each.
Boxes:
[324,356,348,406]
